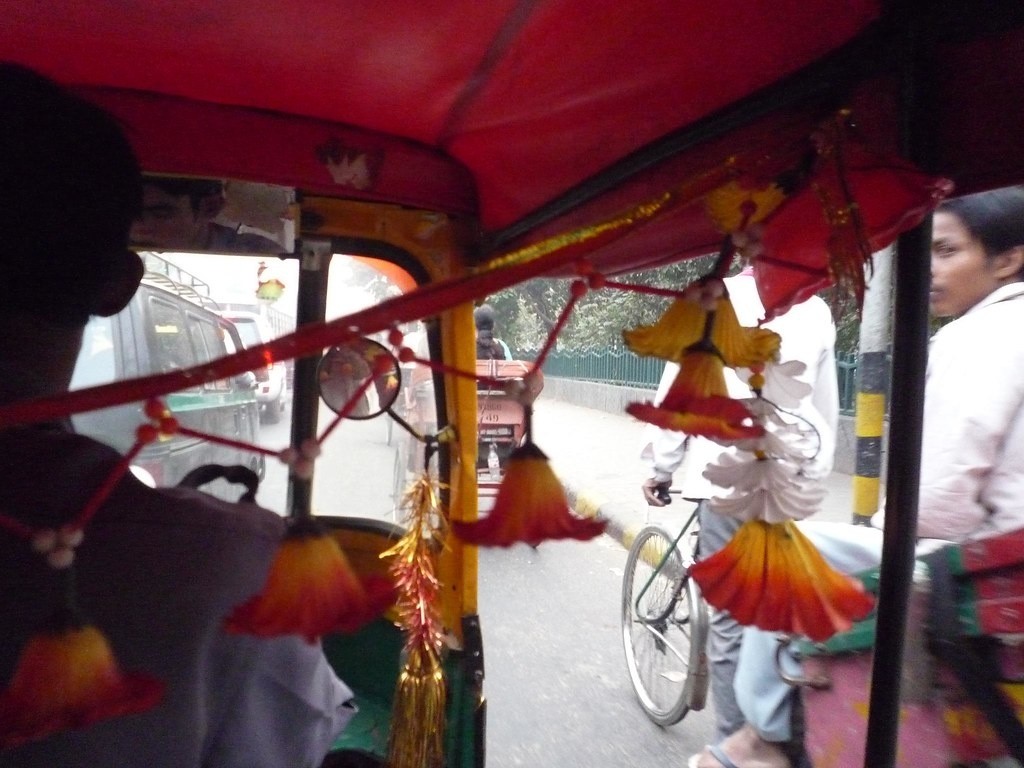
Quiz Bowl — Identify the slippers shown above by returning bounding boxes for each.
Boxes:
[686,746,741,768]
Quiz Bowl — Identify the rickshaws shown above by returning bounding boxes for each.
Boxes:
[386,360,544,529]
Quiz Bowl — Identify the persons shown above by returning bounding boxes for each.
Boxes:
[690,190,1024,768]
[1,59,357,768]
[395,321,420,393]
[474,308,513,474]
[639,251,841,741]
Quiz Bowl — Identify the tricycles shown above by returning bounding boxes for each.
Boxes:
[620,490,1024,768]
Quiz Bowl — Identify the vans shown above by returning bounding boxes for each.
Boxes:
[66,248,265,500]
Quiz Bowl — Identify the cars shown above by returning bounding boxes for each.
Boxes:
[217,311,290,425]
